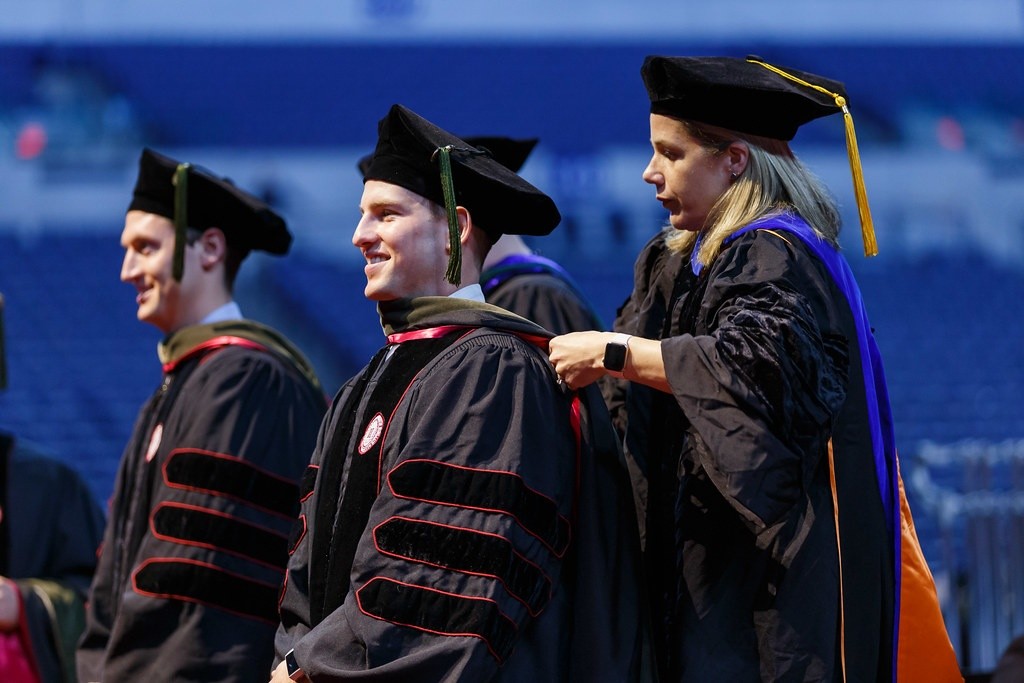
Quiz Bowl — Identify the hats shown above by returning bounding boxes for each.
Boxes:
[359,135,539,173]
[126,148,293,282]
[640,55,877,257]
[363,105,561,285]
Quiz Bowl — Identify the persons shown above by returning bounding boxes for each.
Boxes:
[264,102,584,683]
[442,129,602,338]
[0,295,108,683]
[549,54,897,681]
[76,143,329,683]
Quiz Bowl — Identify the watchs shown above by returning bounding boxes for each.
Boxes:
[602,333,632,381]
[283,648,304,681]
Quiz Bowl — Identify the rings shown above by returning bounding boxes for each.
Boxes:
[555,373,564,385]
